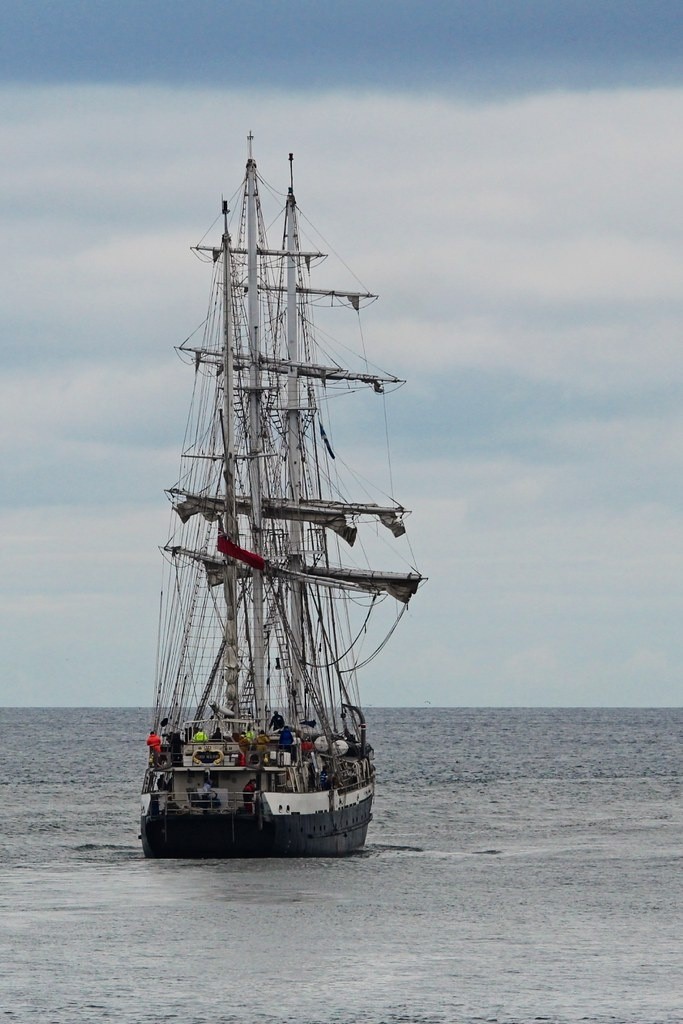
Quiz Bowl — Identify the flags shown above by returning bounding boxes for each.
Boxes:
[217,530,264,569]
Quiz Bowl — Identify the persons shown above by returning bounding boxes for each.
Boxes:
[239,711,293,765]
[243,779,256,815]
[158,774,173,800]
[147,726,221,767]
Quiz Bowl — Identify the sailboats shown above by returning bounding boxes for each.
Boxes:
[138,130,428,859]
